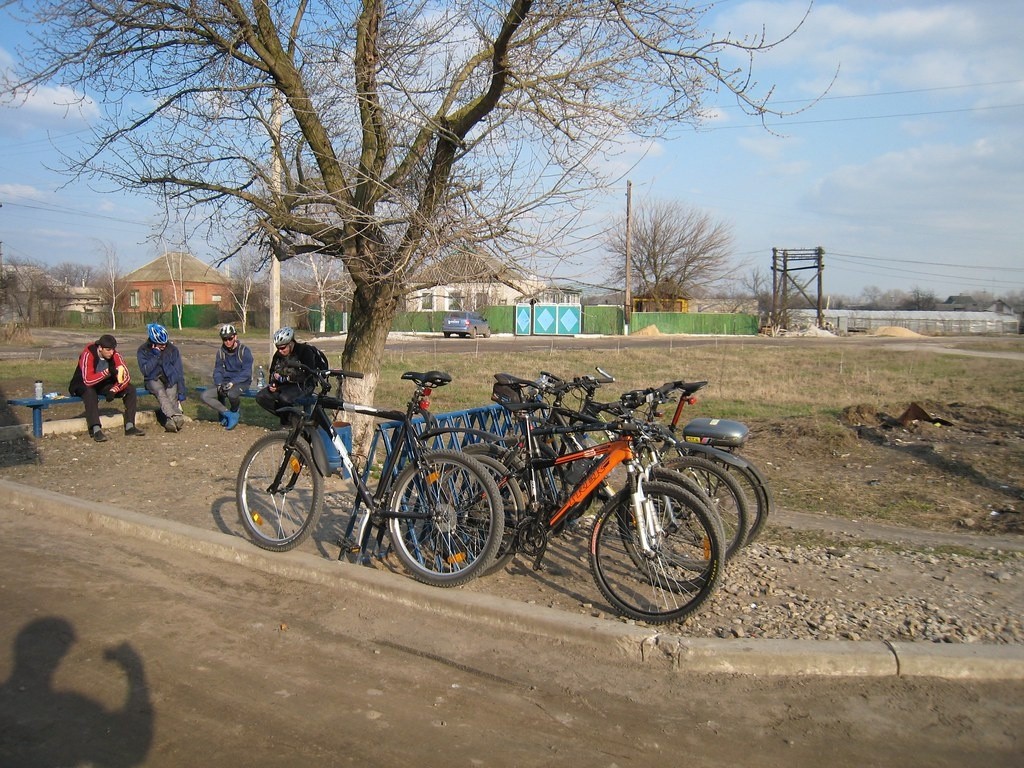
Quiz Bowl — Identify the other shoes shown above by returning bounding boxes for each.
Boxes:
[166,418,177,432]
[172,414,184,428]
[221,410,241,430]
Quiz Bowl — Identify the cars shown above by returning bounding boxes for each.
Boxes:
[442,310,491,340]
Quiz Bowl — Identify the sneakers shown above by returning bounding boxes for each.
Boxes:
[94,431,107,442]
[125,427,145,436]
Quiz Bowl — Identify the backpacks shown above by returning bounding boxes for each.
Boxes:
[299,344,328,379]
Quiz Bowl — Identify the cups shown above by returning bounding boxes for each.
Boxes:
[115,364,124,383]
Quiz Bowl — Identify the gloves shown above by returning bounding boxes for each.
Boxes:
[109,366,118,375]
[103,391,114,402]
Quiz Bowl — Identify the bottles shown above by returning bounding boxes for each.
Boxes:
[558,482,569,507]
[44,392,62,399]
[35,380,43,400]
[583,433,603,459]
[564,456,594,487]
[257,365,265,393]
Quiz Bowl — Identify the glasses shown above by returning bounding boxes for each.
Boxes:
[277,344,289,350]
[223,337,235,341]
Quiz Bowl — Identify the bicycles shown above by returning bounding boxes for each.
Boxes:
[235,361,505,588]
[420,366,772,626]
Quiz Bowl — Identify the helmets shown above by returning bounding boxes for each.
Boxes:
[274,327,294,346]
[147,323,168,344]
[220,325,237,338]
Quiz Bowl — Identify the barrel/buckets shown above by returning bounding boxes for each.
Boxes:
[318,421,352,479]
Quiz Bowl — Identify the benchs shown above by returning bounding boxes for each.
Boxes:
[196,385,318,420]
[6,387,187,438]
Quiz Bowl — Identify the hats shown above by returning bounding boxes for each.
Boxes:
[95,335,117,348]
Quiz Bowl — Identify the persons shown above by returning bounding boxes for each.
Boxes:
[137,323,186,433]
[255,327,328,431]
[68,335,145,442]
[200,324,254,430]
